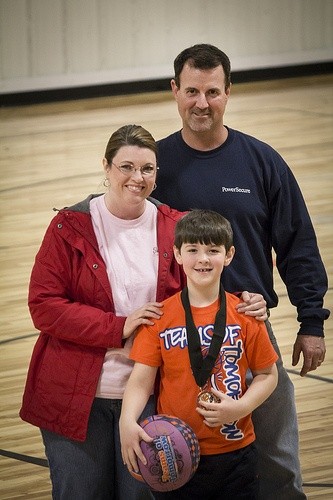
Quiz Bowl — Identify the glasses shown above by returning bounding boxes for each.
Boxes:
[107,159,156,175]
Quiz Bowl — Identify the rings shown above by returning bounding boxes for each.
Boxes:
[318,359,323,363]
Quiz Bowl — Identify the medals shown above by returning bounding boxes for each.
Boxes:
[196,386,219,412]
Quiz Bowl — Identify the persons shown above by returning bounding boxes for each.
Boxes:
[116,208,278,500]
[152,43,330,500]
[17,122,269,500]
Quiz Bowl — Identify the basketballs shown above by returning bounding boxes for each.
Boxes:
[127,414,202,494]
